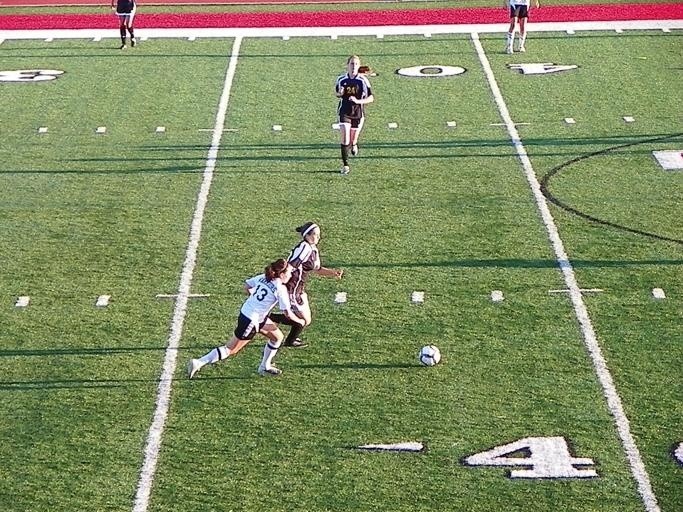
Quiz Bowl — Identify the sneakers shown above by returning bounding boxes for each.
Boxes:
[351,140,358,155]
[283,337,309,349]
[187,358,201,379]
[517,45,526,52]
[120,44,128,49]
[130,38,137,47]
[505,44,513,54]
[258,364,283,377]
[341,165,351,175]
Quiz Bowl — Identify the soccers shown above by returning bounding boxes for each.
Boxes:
[418,345,441,366]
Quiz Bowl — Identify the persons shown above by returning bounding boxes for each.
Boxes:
[334,54,374,175]
[114,0,137,51]
[266,220,342,349]
[187,257,305,379]
[506,1,539,57]
